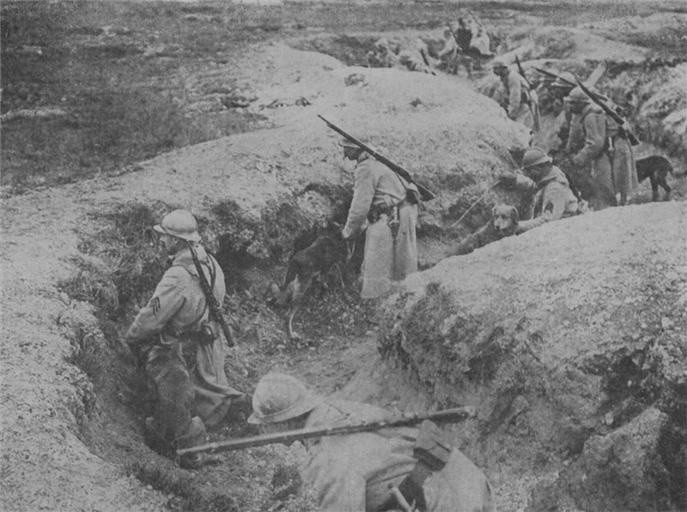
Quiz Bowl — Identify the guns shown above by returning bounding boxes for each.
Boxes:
[420,49,429,67]
[184,238,234,348]
[176,406,477,457]
[571,71,639,146]
[515,56,527,79]
[316,114,436,202]
[531,65,609,102]
[566,175,581,202]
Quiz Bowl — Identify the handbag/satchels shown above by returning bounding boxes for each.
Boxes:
[413,418,454,470]
[202,319,220,342]
[406,183,421,203]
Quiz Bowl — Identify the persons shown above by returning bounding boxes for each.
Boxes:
[367,16,489,81]
[492,61,640,210]
[338,134,418,300]
[125,210,245,427]
[247,373,496,512]
[515,147,584,235]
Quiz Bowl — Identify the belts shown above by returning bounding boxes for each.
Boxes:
[175,330,205,341]
[372,197,414,214]
[373,460,434,512]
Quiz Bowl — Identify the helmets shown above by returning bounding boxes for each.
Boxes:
[338,132,367,150]
[154,209,199,243]
[374,18,592,104]
[248,373,322,425]
[521,148,550,171]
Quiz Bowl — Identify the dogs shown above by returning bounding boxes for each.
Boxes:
[455,203,521,257]
[143,329,208,461]
[270,219,356,341]
[442,53,477,82]
[635,153,675,202]
